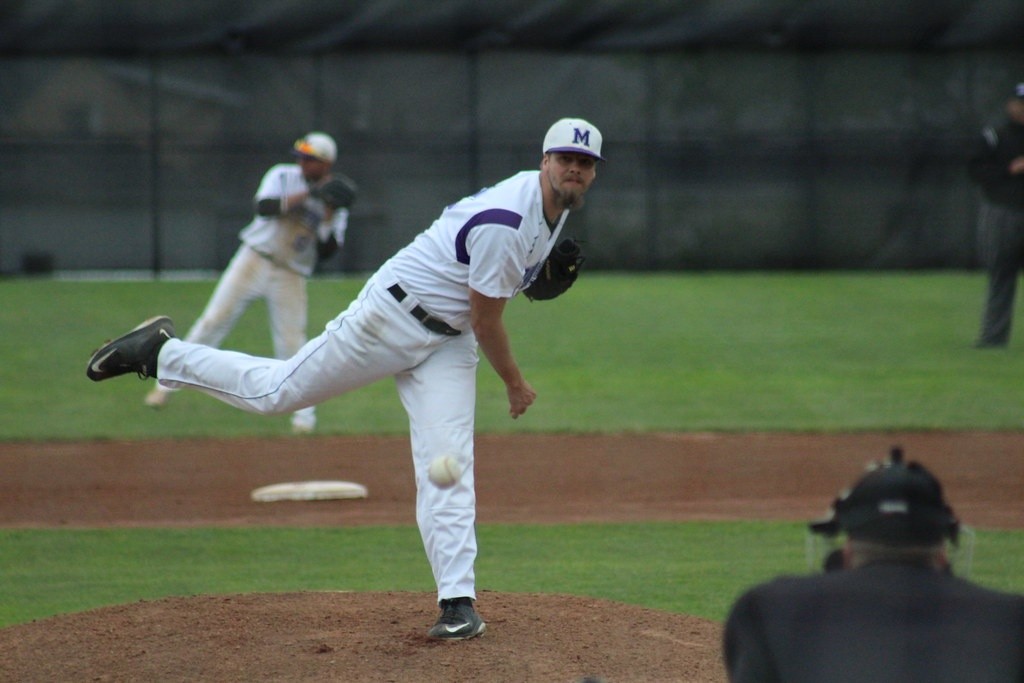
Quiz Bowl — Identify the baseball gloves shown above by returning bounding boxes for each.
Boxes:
[522,235,587,304]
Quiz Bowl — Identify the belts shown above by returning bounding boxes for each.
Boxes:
[387,283,461,336]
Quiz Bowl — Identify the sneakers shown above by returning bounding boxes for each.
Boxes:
[87,315,176,382]
[426,597,486,641]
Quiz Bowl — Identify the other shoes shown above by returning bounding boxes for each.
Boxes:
[145,388,171,406]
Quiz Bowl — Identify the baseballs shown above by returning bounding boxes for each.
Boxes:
[426,455,460,489]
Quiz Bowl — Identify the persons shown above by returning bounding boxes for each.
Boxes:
[970,83,1024,349]
[723,447,1024,683]
[84,112,604,644]
[142,132,358,437]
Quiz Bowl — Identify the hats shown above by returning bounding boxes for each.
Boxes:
[543,118,607,163]
[840,464,946,547]
[288,132,337,165]
